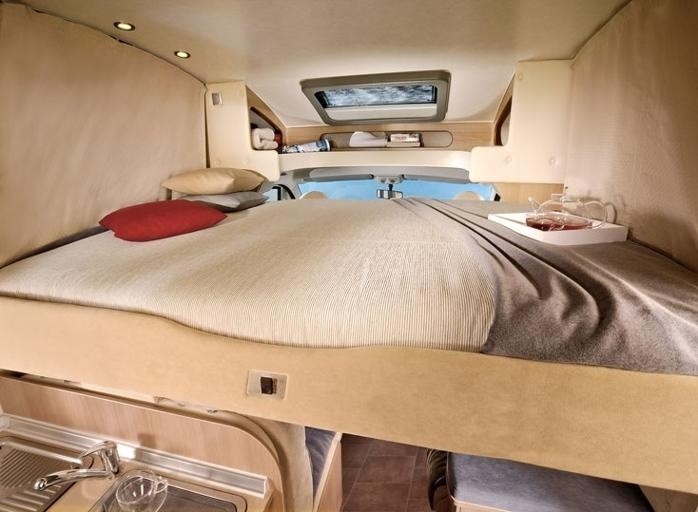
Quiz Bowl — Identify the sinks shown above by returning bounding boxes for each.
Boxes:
[0,434,93,512]
[89,467,248,512]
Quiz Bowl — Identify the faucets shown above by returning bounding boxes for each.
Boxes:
[32,440,122,491]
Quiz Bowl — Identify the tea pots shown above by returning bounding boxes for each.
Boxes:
[528,193,608,231]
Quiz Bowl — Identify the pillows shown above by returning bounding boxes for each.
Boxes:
[172,192,268,212]
[99,200,227,241]
[161,168,264,196]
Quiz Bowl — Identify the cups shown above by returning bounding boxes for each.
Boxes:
[525,213,565,231]
[116,473,167,511]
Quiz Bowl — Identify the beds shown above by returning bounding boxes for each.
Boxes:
[1,196,698,508]
